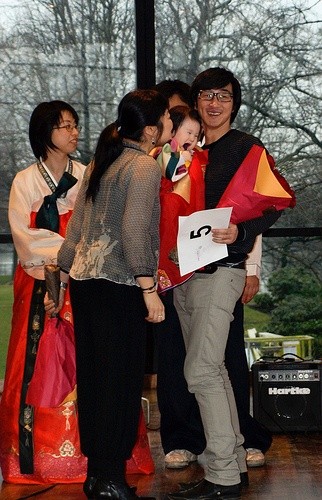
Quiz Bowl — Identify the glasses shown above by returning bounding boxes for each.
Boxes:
[198,90,234,102]
[53,124,79,133]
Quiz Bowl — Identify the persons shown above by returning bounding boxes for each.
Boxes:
[44,89,173,500]
[0,99,156,486]
[167,66,296,500]
[142,79,274,470]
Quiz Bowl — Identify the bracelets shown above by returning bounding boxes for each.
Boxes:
[141,286,155,290]
[59,280,68,290]
[142,290,156,294]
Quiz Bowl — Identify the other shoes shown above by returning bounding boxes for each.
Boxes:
[246,448,265,467]
[177,472,250,491]
[83,475,156,500]
[164,449,196,468]
[167,478,242,500]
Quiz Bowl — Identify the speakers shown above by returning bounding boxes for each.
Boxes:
[251,363,322,435]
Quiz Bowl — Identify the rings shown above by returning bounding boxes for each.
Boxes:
[158,315,163,318]
